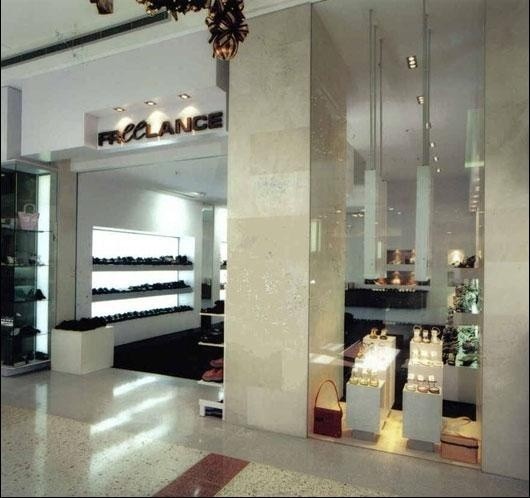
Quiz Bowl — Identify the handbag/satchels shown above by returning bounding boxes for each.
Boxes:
[440,433,479,465]
[313,379,343,439]
[17,202,40,231]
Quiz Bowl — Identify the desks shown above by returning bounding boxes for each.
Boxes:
[50,324,115,375]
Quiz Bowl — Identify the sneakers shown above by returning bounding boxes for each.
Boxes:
[202,357,224,382]
[200,321,223,344]
[92,255,194,324]
[200,299,224,314]
[3,289,49,367]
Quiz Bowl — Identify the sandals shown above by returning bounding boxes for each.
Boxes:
[405,324,442,394]
[348,328,389,388]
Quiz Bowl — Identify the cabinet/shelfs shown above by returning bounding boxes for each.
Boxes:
[0,158,60,378]
[198,312,225,421]
[92,262,194,324]
[442,267,479,406]
